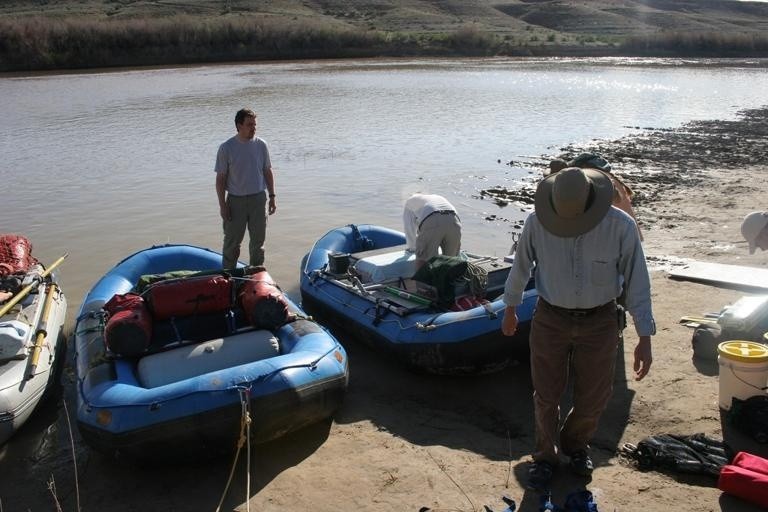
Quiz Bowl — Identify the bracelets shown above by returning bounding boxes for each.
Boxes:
[269,194,276,198]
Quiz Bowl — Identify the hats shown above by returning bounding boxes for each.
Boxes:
[535,167,613,237]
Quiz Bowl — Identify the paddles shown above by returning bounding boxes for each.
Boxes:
[28,267,62,379]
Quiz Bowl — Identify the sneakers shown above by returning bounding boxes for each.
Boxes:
[528,462,552,491]
[569,449,594,475]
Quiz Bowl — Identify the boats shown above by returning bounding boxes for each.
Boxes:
[69,243,351,460]
[298,223,542,380]
[0,264,63,442]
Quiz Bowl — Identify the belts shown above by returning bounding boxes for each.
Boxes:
[552,305,599,316]
[419,210,459,230]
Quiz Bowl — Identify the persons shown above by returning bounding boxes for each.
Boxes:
[214,108,276,270]
[403,194,462,272]
[568,152,644,243]
[502,167,657,492]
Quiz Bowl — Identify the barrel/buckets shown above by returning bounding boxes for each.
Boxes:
[716,340,767,412]
